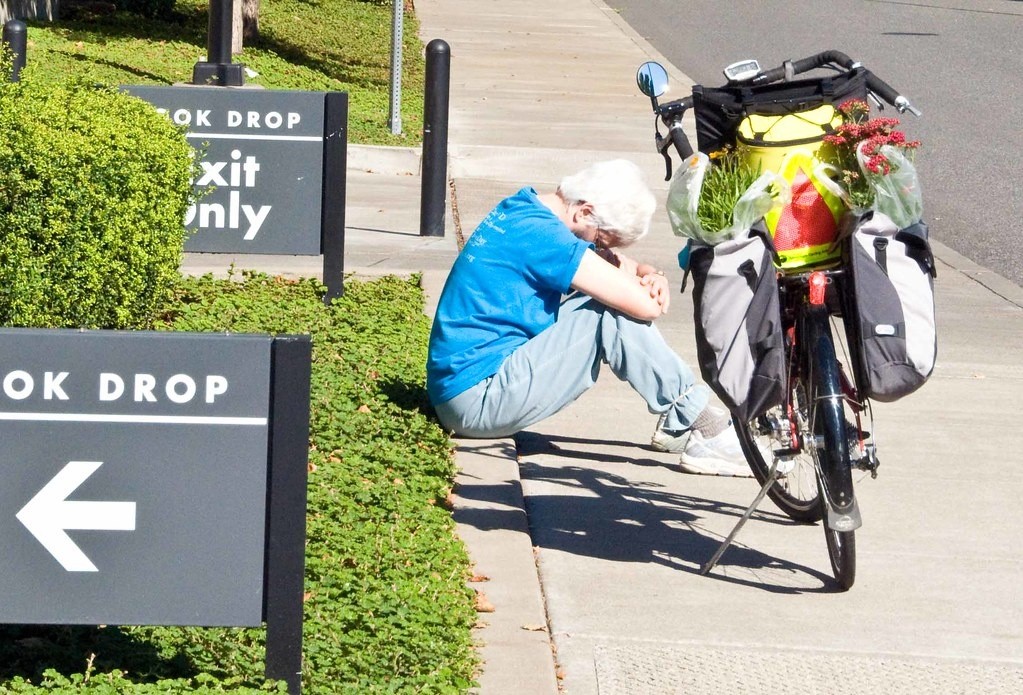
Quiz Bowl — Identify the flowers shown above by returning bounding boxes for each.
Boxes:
[823,97,922,228]
[689,147,780,241]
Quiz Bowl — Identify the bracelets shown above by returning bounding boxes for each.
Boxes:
[655,271,666,277]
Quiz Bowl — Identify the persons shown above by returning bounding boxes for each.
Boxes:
[426,159,793,480]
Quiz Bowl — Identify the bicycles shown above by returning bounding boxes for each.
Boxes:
[636,48,925,590]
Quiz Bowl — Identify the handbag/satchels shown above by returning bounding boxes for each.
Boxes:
[691,68,868,167]
[677,217,789,418]
[831,210,937,403]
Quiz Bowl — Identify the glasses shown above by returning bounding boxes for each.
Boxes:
[595,225,604,251]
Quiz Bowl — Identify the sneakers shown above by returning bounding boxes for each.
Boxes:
[679,419,795,478]
[651,412,691,453]
